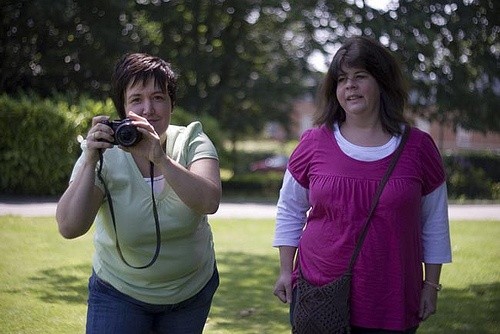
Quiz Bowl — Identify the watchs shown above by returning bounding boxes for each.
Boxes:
[423,280,443,291]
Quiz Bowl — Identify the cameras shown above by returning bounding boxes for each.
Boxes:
[100,119,143,148]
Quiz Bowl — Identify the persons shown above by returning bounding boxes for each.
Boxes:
[55,53,222,334]
[271,35,453,334]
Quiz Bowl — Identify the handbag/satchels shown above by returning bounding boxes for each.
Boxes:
[292,275,353,334]
